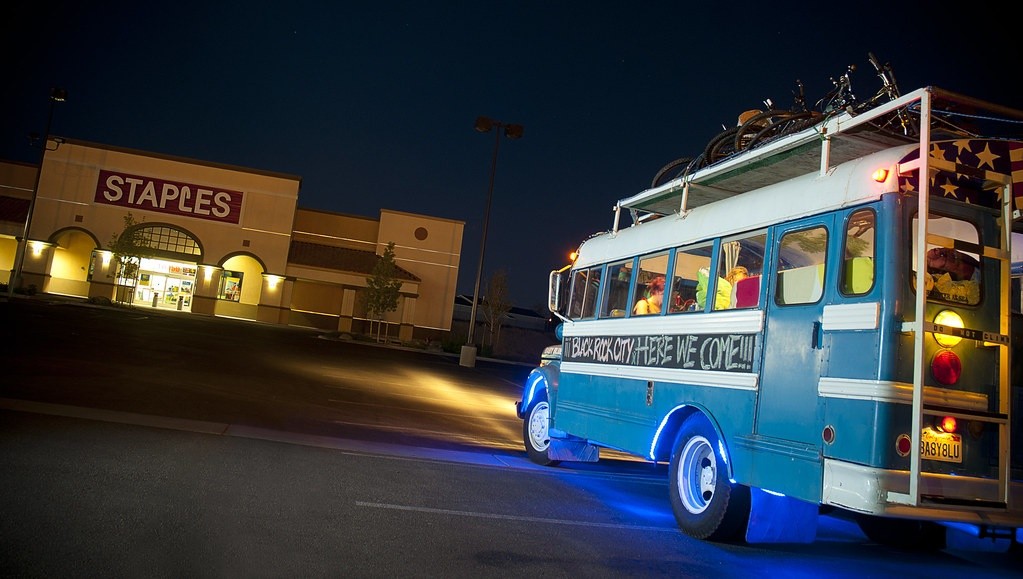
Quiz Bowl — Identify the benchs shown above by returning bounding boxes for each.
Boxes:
[729,257,873,309]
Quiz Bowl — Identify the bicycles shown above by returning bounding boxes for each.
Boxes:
[649,54,921,190]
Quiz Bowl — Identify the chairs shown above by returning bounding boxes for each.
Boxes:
[610,309,626,318]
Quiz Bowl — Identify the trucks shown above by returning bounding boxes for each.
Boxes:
[513,85,1023,557]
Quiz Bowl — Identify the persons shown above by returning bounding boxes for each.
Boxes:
[633,277,666,315]
[725,266,748,286]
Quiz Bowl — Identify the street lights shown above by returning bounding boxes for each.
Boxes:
[459,115,523,370]
